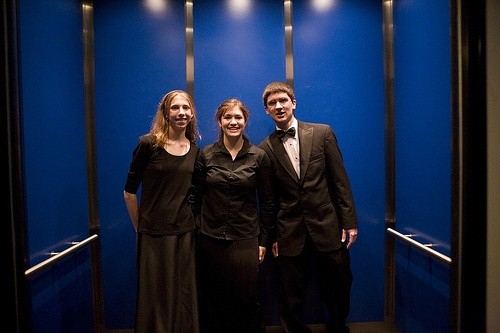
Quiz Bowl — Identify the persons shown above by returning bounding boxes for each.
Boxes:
[258,81,359,333]
[201,98,266,333]
[122,90,199,333]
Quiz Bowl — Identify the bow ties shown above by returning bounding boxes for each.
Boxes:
[275,127,295,140]
